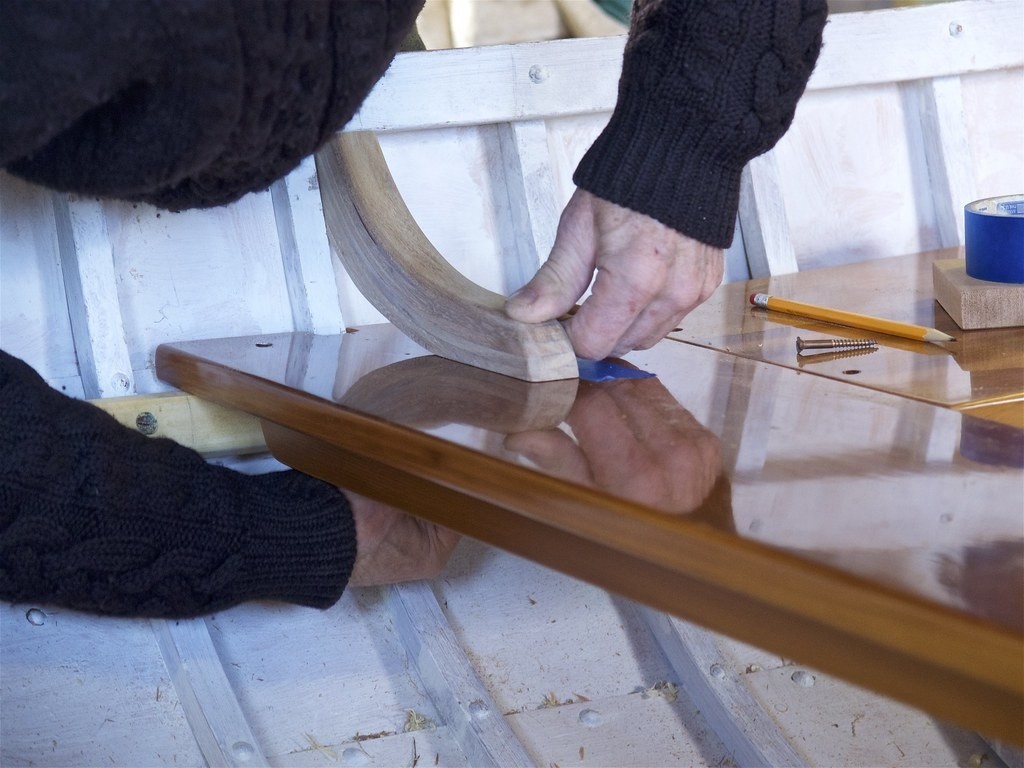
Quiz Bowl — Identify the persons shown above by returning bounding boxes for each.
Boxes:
[0,0,828,622]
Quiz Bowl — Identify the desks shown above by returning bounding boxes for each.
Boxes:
[143,235,1024,763]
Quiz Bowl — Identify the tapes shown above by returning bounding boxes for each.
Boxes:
[964,193,1023,283]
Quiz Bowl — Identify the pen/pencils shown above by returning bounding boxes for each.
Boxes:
[749,293,958,343]
